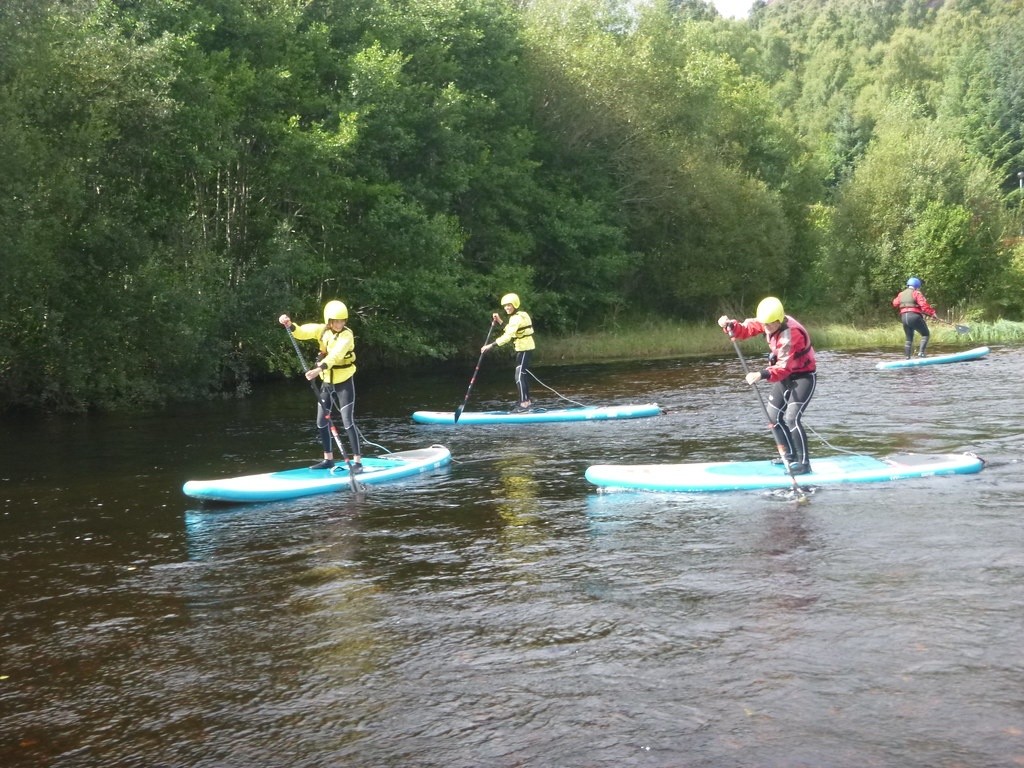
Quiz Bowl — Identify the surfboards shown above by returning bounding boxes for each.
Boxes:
[875,346,990,369]
[413,405,661,424]
[584,453,983,494]
[182,447,451,504]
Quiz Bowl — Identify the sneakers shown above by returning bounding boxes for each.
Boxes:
[771,456,798,464]
[785,462,812,475]
[350,462,363,474]
[309,459,334,469]
[510,406,533,414]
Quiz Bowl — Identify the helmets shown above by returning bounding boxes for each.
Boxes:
[501,293,520,309]
[907,277,921,289]
[324,301,349,324]
[757,297,784,323]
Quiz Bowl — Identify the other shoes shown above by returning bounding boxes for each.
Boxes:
[906,357,911,360]
[918,354,929,358]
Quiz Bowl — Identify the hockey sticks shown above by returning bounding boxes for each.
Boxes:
[453,316,498,423]
[922,312,970,334]
[723,323,807,498]
[284,321,368,493]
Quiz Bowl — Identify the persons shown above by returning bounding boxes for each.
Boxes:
[480,294,536,414]
[892,278,937,360]
[279,300,364,474]
[718,296,817,475]
